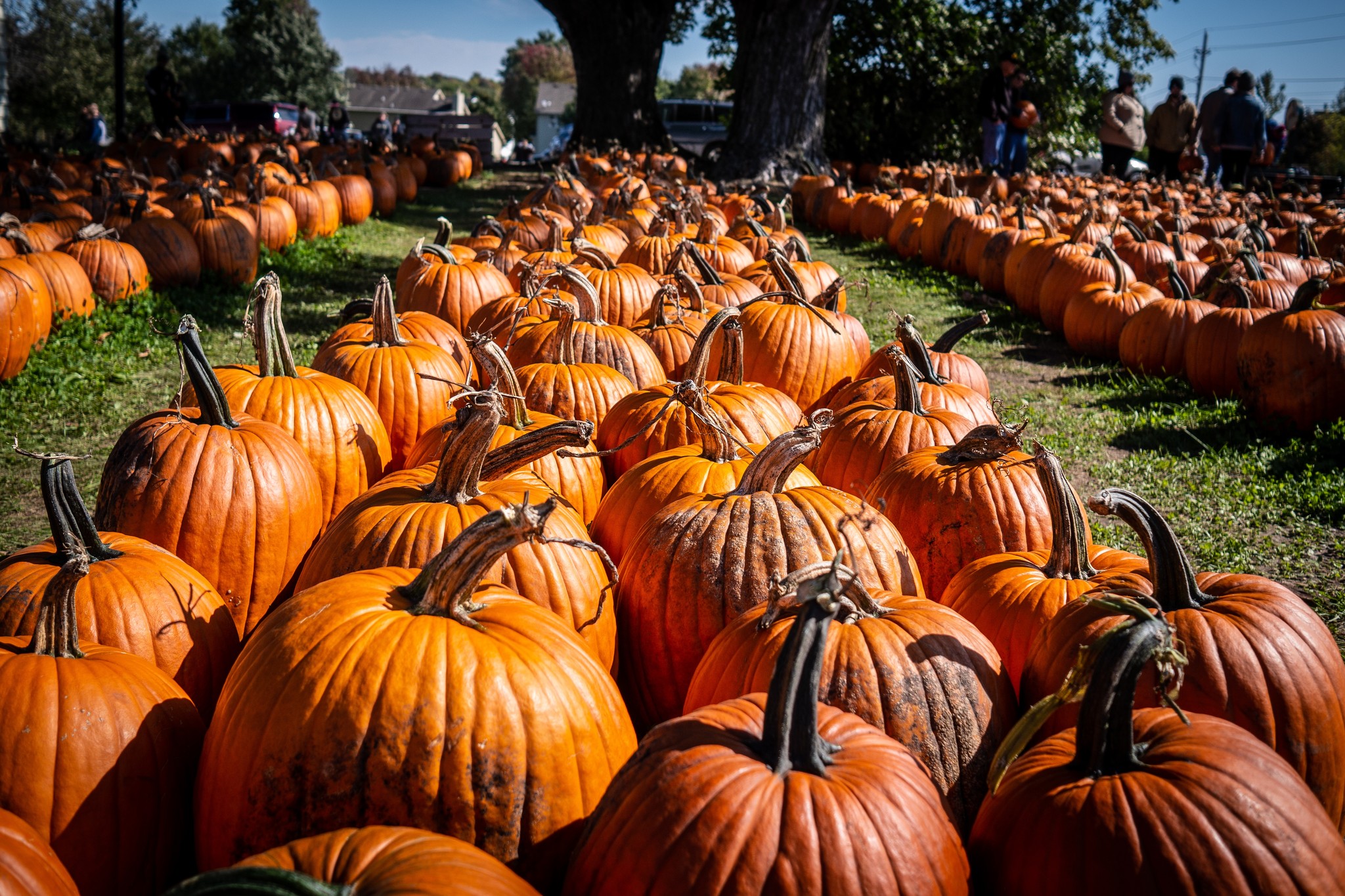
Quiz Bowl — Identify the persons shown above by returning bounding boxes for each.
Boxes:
[73,56,1338,207]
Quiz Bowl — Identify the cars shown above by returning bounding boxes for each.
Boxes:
[1044,143,1151,183]
[657,100,741,163]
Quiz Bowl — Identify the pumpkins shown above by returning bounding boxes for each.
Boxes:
[0,130,1344,896]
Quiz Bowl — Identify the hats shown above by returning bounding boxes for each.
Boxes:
[1238,71,1256,92]
[1169,78,1184,90]
